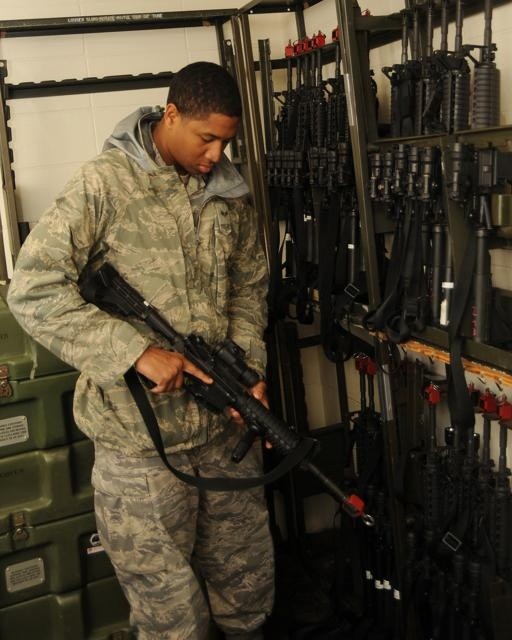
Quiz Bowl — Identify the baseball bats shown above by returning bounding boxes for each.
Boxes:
[392,355,510,639]
[303,331,400,637]
[75,241,376,531]
[265,8,367,365]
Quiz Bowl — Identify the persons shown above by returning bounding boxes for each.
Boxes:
[4,60,279,640]
